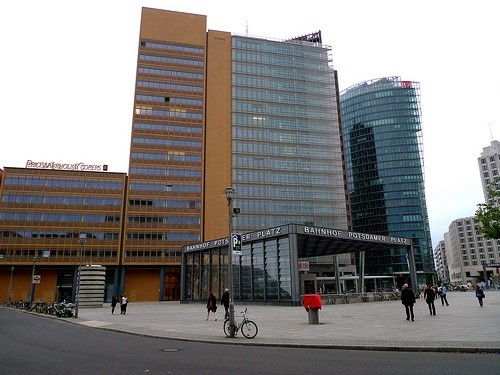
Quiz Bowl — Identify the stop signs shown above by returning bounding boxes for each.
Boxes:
[34,277,39,282]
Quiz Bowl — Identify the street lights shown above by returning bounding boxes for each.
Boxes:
[29,256,38,302]
[75,233,86,319]
[8,267,14,300]
[225,187,240,338]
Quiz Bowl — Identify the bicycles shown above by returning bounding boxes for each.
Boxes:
[223,308,258,339]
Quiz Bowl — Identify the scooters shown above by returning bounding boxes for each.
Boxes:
[2,300,77,317]
[326,291,420,304]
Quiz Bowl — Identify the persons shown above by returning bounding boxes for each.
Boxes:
[401,283,416,322]
[423,285,436,316]
[206,292,218,321]
[431,286,442,299]
[476,286,485,307]
[120,292,129,315]
[111,294,117,315]
[457,286,466,292]
[221,289,230,319]
[439,283,449,306]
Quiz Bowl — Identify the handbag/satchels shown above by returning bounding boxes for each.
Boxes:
[481,294,485,298]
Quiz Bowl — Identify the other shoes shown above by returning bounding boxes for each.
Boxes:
[406,318,410,320]
[411,319,414,322]
